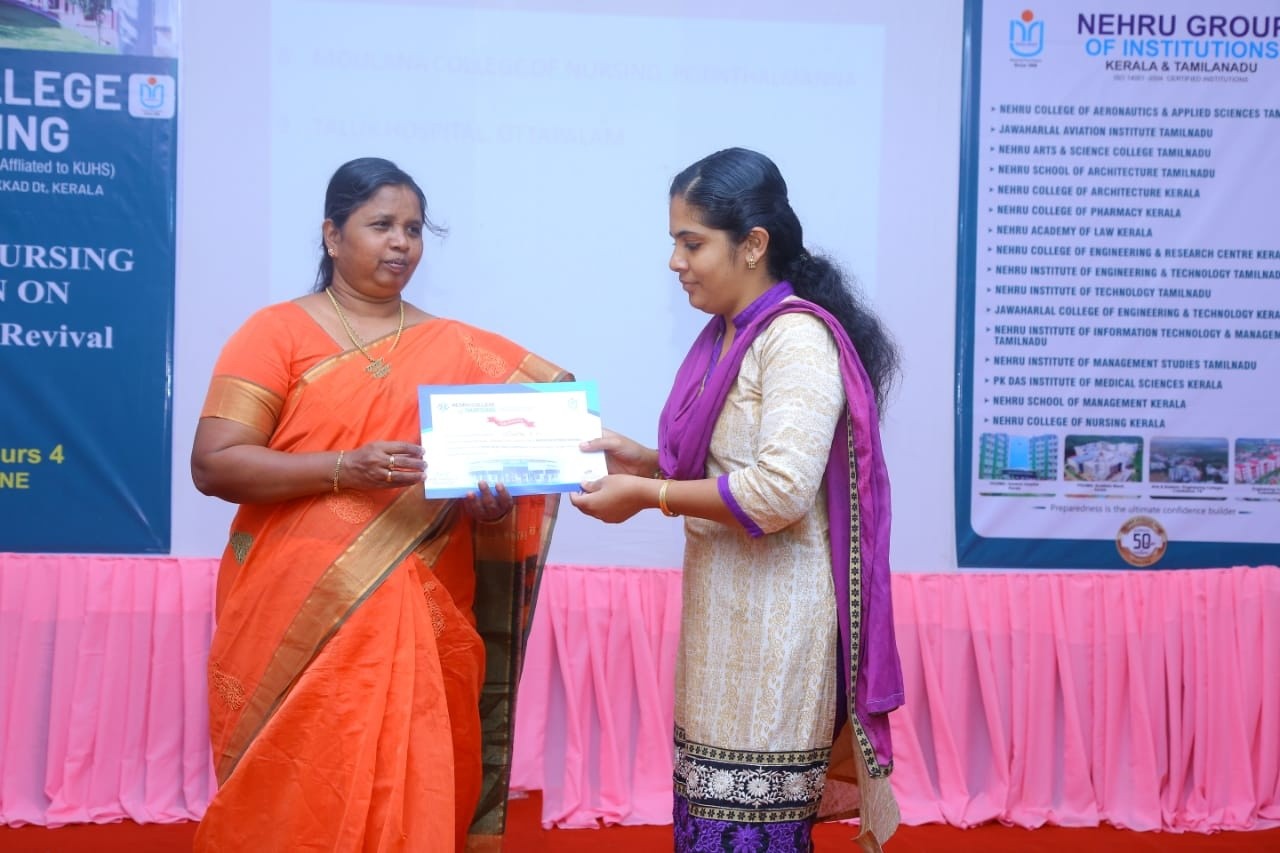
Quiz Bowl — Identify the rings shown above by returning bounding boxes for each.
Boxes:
[390,455,394,469]
[387,471,392,482]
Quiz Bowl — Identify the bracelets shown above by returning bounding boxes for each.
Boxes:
[654,447,663,479]
[335,451,344,493]
[659,479,680,517]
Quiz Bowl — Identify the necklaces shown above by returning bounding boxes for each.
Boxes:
[323,287,404,363]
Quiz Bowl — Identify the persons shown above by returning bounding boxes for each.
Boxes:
[192,159,575,853]
[569,146,903,853]
